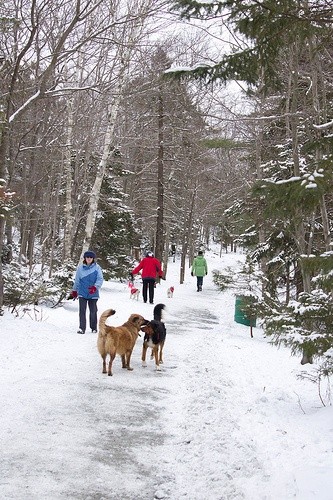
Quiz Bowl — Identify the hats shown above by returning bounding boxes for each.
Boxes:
[198,251,203,255]
[84,251,95,259]
[146,252,154,258]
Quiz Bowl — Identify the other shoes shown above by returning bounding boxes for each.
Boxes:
[78,330,85,334]
[92,329,96,333]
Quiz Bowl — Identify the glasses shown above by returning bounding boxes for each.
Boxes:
[86,256,92,258]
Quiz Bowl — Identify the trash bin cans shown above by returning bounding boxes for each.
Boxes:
[234,294,258,328]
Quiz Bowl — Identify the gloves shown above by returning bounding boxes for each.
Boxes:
[88,286,96,294]
[191,272,193,276]
[206,272,207,275]
[73,291,77,297]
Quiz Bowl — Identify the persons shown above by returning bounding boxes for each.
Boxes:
[131,251,163,303]
[191,251,207,292]
[72,251,103,334]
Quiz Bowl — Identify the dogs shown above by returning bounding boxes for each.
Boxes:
[139,302,168,369]
[96,308,149,377]
[126,279,134,289]
[129,288,141,302]
[166,285,175,299]
